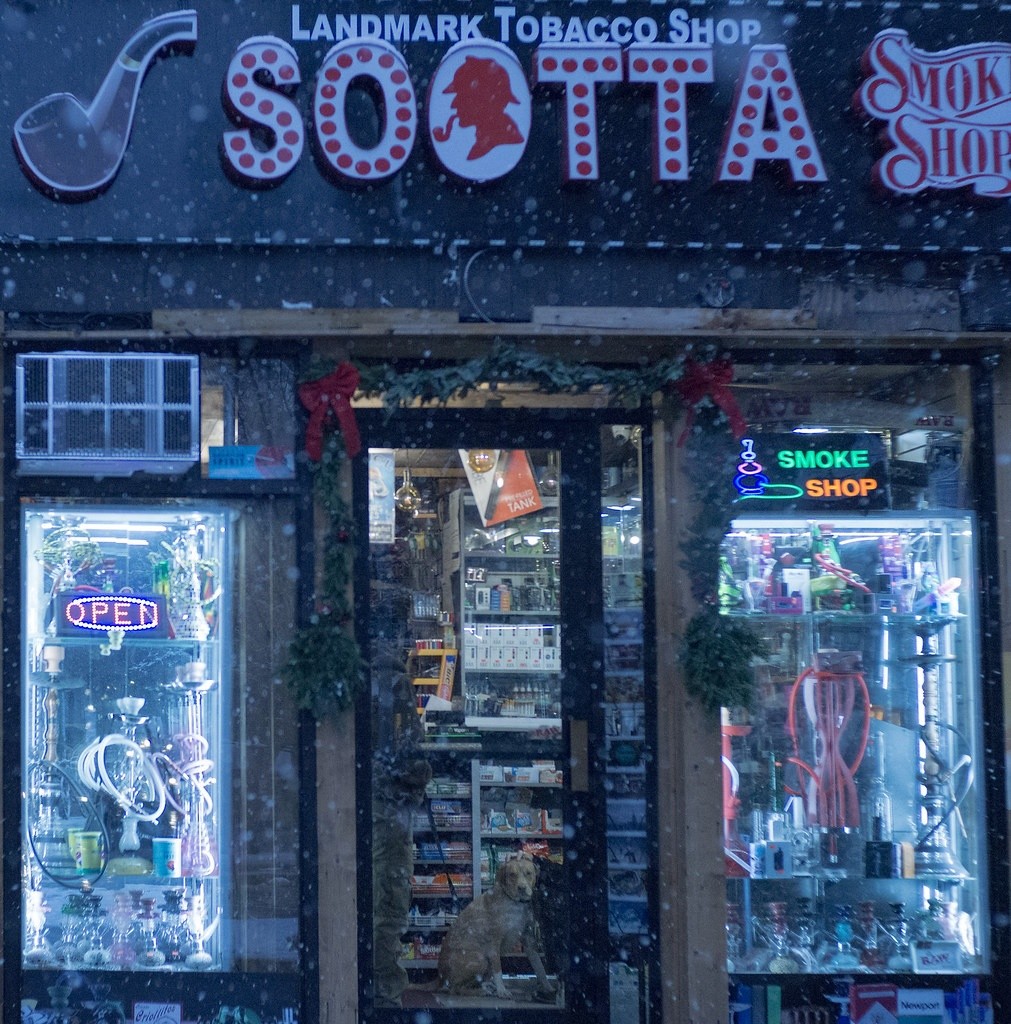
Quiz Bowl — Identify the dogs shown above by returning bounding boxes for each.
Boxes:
[407,858,558,1000]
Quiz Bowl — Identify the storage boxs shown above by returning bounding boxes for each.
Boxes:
[461,623,561,670]
[397,770,471,960]
[783,569,811,614]
[482,760,563,886]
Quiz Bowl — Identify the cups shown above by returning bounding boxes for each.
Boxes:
[152,838,183,877]
[67,827,84,858]
[76,832,102,871]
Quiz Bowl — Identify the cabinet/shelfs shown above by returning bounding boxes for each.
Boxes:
[19,502,232,973]
[437,486,562,728]
[606,608,647,936]
[469,758,562,959]
[398,743,483,972]
[715,509,990,974]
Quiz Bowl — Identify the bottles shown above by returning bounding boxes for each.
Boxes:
[867,777,894,842]
